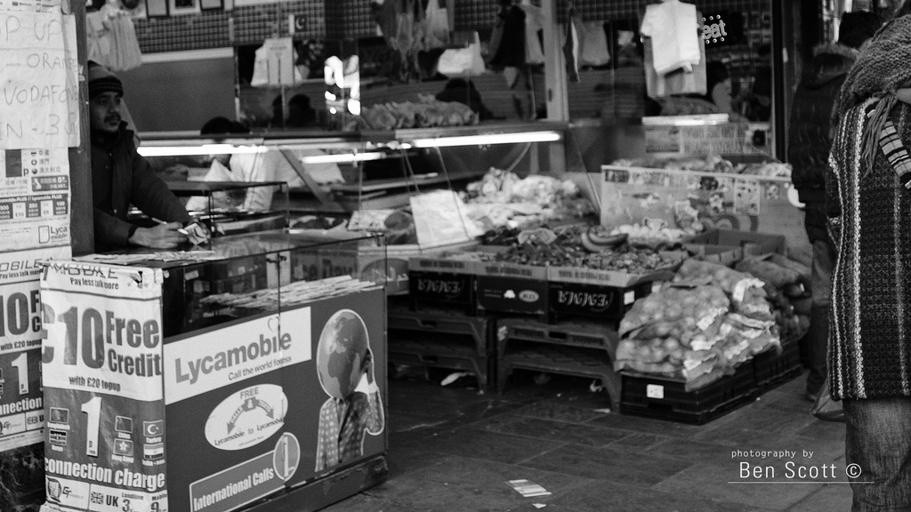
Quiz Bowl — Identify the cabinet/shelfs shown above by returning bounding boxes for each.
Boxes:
[40,226,393,512]
[137,119,601,262]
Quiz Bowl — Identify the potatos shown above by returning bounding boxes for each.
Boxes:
[616,259,779,379]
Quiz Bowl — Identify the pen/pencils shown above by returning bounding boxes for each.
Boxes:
[151,217,190,235]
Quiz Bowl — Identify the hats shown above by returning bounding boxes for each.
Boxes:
[87,60,125,94]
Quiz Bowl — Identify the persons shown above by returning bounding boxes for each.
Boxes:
[783,10,877,421]
[286,93,317,126]
[824,1,911,512]
[312,348,386,473]
[87,57,212,250]
[185,116,347,217]
